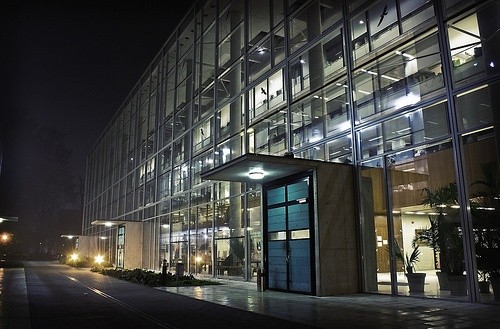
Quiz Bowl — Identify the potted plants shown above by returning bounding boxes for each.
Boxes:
[412,180,500,299]
[385,242,426,293]
